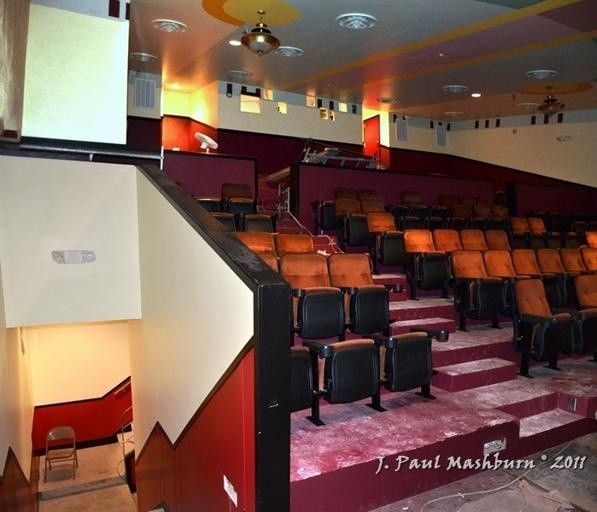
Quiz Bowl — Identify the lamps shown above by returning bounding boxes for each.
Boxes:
[536,84,565,118]
[242,9,281,59]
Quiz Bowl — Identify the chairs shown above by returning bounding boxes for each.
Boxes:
[43,425,79,484]
[191,182,596,426]
